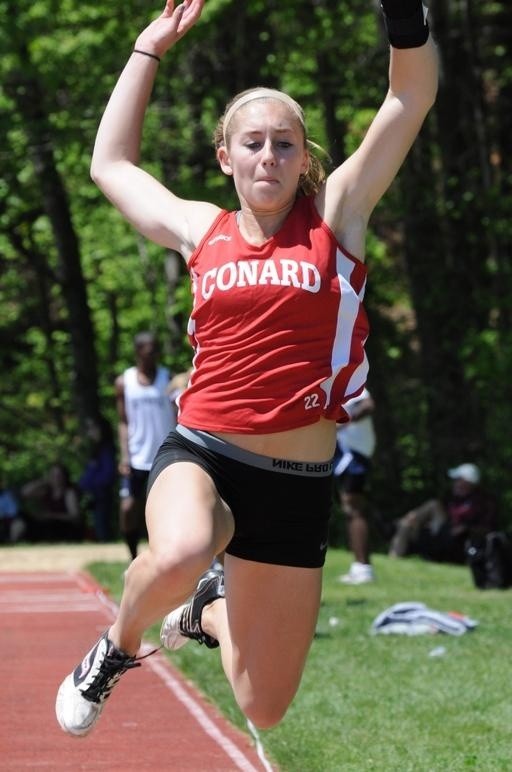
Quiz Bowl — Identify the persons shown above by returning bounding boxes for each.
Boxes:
[1,468,26,545]
[51,0,443,738]
[114,328,179,562]
[77,412,118,542]
[335,388,381,585]
[388,460,488,562]
[21,462,89,543]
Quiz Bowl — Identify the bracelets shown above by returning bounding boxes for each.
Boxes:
[379,0,431,50]
[131,46,162,67]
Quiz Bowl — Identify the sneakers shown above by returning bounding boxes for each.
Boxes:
[337,562,374,586]
[160,569,226,651]
[55,625,143,737]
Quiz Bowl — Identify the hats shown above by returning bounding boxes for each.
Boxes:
[448,463,481,484]
